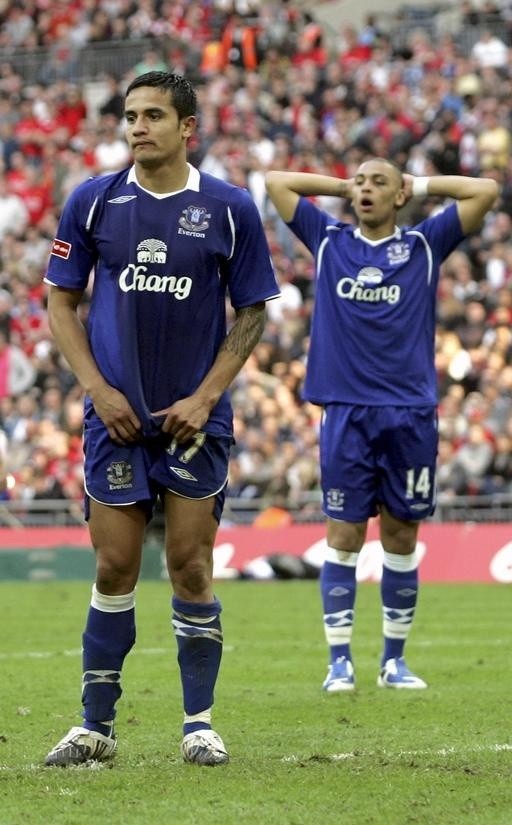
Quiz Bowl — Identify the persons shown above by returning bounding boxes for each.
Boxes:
[38,70,281,775]
[0,1,512,529]
[265,154,500,698]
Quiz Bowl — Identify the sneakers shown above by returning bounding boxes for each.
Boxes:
[181,729,229,766]
[376,655,428,690]
[323,656,355,694]
[44,725,119,765]
